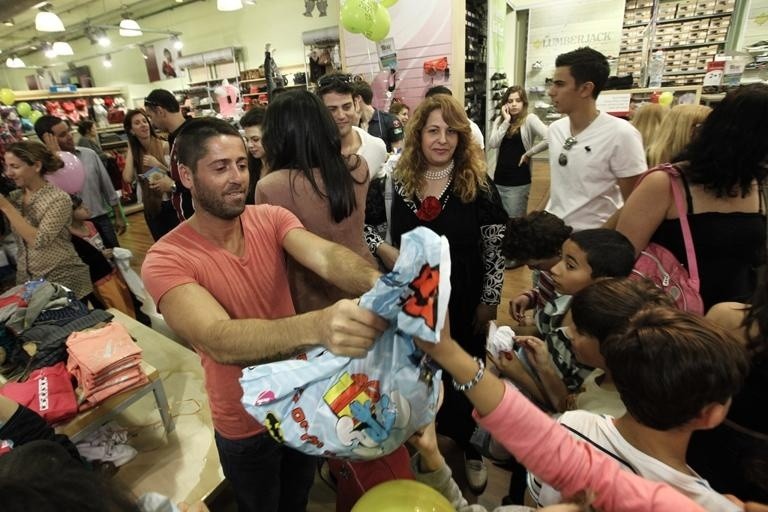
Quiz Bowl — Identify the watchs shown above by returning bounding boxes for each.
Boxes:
[170,181,177,193]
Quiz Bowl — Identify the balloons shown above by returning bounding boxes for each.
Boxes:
[18,103,30,117]
[365,5,391,42]
[26,108,41,125]
[381,1,399,9]
[339,0,378,34]
[2,88,14,106]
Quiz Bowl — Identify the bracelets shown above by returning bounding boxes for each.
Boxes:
[451,357,483,390]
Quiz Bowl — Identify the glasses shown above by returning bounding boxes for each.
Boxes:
[316,77,350,86]
[144,97,162,106]
[559,137,577,166]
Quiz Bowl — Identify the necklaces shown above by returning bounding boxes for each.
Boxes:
[423,159,457,181]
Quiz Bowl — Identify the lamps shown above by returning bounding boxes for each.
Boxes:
[119,4,143,37]
[6,52,26,68]
[35,3,66,32]
[171,33,184,50]
[47,35,74,56]
[92,27,112,48]
[103,54,112,68]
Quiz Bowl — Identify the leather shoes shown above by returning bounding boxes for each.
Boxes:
[464,452,488,493]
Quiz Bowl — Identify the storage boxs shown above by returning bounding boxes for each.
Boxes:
[723,75,741,83]
[725,61,745,74]
[707,62,724,71]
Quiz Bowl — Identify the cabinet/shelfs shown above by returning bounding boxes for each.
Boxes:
[601,85,702,104]
[14,86,144,214]
[526,117,555,214]
[302,26,346,92]
[452,0,486,154]
[616,0,750,88]
[240,75,283,111]
[176,46,242,113]
[54,362,175,442]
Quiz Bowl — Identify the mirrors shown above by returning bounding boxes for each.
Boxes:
[101,140,144,215]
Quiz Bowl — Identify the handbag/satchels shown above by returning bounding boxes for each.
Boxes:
[626,164,704,317]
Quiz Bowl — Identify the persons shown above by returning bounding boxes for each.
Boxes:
[353,81,405,154]
[142,117,384,511]
[144,91,195,224]
[0,439,177,512]
[123,109,178,242]
[616,82,766,504]
[161,46,179,78]
[77,120,111,164]
[651,105,712,178]
[428,84,486,151]
[254,89,378,318]
[535,228,637,389]
[488,85,550,269]
[0,393,57,450]
[308,48,329,85]
[411,379,598,512]
[34,115,127,264]
[235,109,272,205]
[391,104,409,130]
[0,141,97,311]
[69,195,149,329]
[493,278,678,419]
[505,209,573,325]
[532,49,647,284]
[634,106,670,152]
[363,93,510,489]
[320,75,387,182]
[415,308,766,511]
